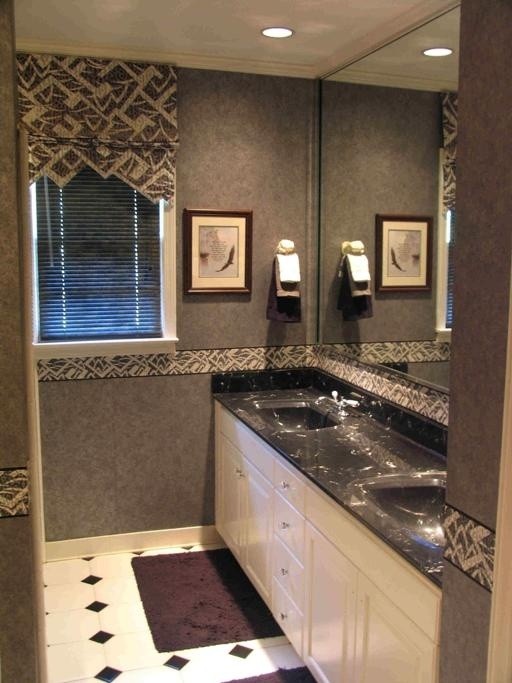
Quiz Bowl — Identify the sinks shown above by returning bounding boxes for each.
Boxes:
[360,479,449,560]
[251,397,343,433]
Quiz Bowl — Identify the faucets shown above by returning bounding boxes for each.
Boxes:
[313,391,360,416]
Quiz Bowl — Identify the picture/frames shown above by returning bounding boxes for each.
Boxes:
[377,213,435,294]
[182,208,253,293]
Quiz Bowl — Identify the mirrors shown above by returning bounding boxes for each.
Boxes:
[317,8,464,399]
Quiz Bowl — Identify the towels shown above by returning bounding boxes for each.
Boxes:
[274,252,303,300]
[342,252,374,298]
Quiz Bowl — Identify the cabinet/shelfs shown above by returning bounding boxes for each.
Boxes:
[306,487,442,683]
[219,407,275,605]
[270,458,307,660]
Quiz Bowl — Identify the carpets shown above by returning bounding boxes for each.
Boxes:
[129,546,284,653]
